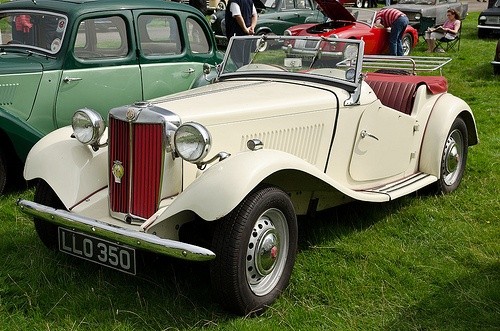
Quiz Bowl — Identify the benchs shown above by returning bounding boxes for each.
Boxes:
[297,69,445,113]
[141,43,176,56]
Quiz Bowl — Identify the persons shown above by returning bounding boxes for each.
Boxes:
[371,8,409,56]
[225,0,258,70]
[16,15,33,41]
[425,9,461,52]
[189,0,207,43]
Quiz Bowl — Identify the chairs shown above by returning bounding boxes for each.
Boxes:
[433,21,462,52]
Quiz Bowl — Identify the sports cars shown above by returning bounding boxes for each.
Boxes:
[17,35,480,320]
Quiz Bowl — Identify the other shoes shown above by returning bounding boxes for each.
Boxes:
[435,49,445,53]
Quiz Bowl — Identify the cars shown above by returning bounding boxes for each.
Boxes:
[76,0,500,76]
[0,0,239,198]
